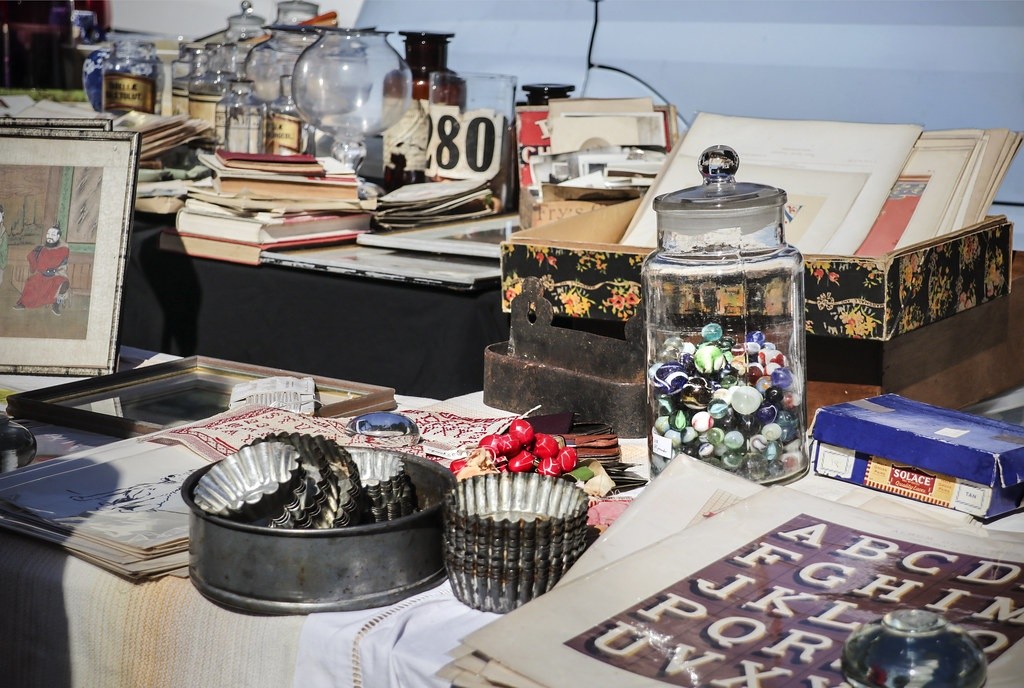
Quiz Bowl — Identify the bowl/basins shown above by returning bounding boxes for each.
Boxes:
[181,447,453,616]
[841,608,986,688]
[440,471,589,615]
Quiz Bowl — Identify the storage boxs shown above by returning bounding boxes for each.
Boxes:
[499,98,1024,532]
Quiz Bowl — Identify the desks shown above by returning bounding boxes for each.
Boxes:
[118,212,512,403]
[0,343,1024,688]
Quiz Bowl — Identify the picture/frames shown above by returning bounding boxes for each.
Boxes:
[5,354,399,446]
[0,115,142,382]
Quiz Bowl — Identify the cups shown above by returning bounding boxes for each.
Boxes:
[427,71,518,209]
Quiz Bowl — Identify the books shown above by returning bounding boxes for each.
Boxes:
[0,93,378,266]
[0,419,214,583]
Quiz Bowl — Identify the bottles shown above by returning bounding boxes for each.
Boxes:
[645,146,811,485]
[515,82,575,112]
[80,0,415,201]
[381,31,466,192]
